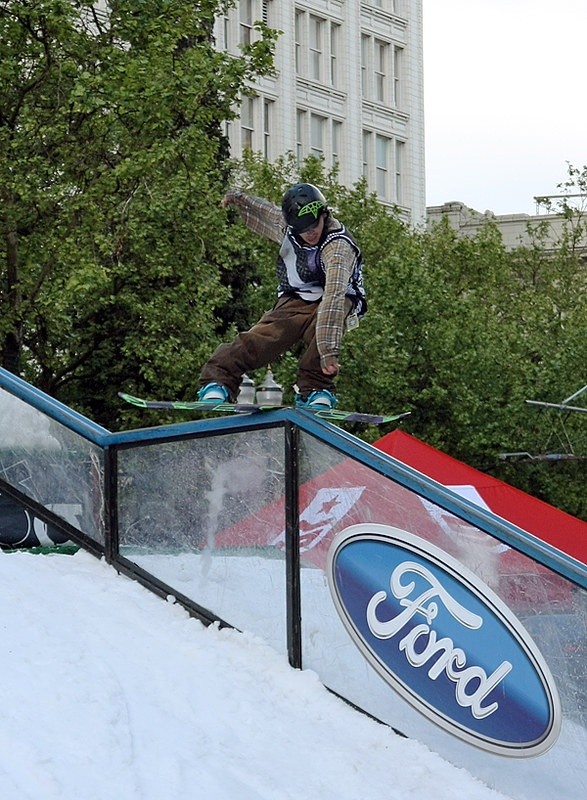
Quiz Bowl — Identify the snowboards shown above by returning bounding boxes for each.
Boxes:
[119,392,412,423]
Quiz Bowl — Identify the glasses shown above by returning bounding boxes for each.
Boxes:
[298,218,320,233]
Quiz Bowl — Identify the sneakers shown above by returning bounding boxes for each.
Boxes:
[308,389,332,407]
[196,380,228,403]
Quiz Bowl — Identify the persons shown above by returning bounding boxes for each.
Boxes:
[194,181,368,412]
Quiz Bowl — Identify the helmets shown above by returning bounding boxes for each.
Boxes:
[281,183,328,230]
